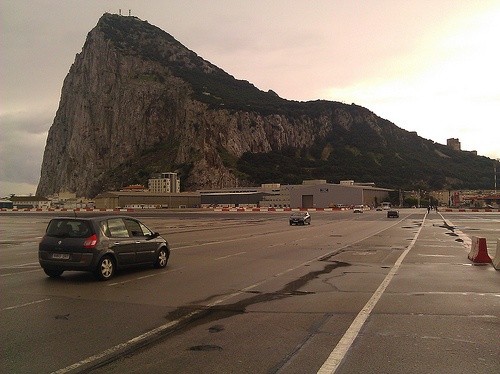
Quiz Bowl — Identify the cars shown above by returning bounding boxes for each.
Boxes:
[38,209,170,281]
[289,211,312,226]
[376,206,382,211]
[387,209,400,218]
[384,206,390,210]
[353,205,364,213]
